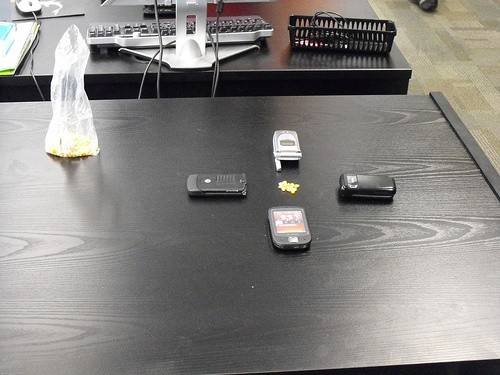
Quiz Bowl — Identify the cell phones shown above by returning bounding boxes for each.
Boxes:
[338,173,398,201]
[266,205,312,253]
[187,172,248,197]
[271,130,303,172]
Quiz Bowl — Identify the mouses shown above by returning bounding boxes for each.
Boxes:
[14,0,41,13]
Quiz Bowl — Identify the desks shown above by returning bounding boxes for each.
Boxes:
[2,90,500,375]
[0,0,413,102]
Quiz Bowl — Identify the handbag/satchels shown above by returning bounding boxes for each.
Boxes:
[44,24,101,158]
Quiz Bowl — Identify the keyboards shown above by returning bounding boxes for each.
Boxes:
[85,14,274,54]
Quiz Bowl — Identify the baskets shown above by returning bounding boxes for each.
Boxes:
[288,13,397,55]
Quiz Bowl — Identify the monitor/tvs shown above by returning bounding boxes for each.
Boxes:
[99,0,273,6]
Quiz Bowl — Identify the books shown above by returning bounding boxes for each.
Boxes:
[0,22,18,57]
